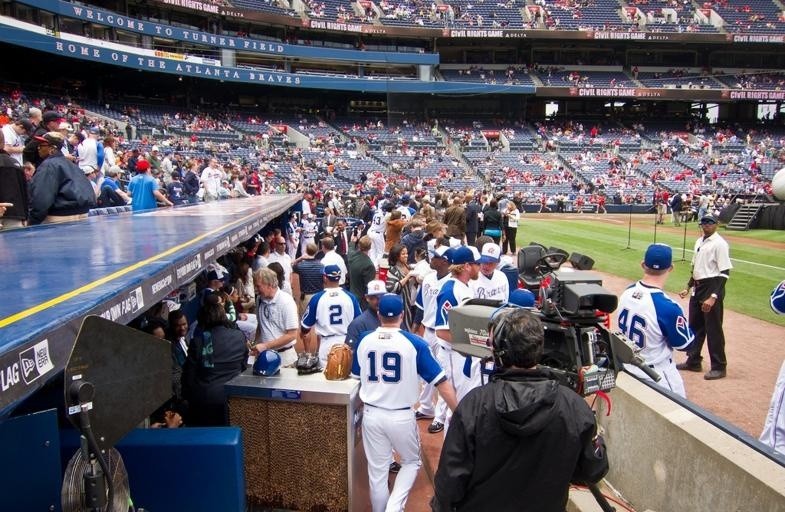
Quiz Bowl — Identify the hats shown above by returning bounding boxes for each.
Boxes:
[481,243,500,263]
[378,293,403,317]
[83,166,99,175]
[75,131,85,146]
[320,265,341,277]
[644,243,672,269]
[402,194,412,200]
[508,289,535,310]
[136,160,150,170]
[161,301,181,321]
[152,145,158,152]
[33,131,64,144]
[42,111,63,121]
[700,212,718,223]
[90,126,100,135]
[428,246,455,263]
[108,165,125,176]
[453,246,489,264]
[207,266,230,281]
[365,280,388,296]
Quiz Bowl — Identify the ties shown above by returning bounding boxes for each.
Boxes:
[341,232,346,254]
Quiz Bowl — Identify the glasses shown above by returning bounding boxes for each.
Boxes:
[278,242,286,245]
[701,220,715,225]
[38,143,49,148]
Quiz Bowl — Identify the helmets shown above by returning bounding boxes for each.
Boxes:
[253,350,281,376]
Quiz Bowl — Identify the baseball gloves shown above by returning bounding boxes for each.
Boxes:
[326,344,354,380]
[297,353,321,375]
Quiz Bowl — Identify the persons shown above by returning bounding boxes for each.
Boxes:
[303,109,520,256]
[428,311,610,512]
[250,267,300,368]
[345,279,408,376]
[470,243,510,303]
[617,243,696,401]
[364,255,385,279]
[300,265,325,355]
[385,244,421,339]
[454,61,785,96]
[710,293,718,299]
[267,0,785,37]
[759,272,785,457]
[434,246,480,440]
[269,267,300,305]
[410,247,452,434]
[258,209,363,267]
[675,213,734,379]
[132,230,258,427]
[1,89,301,232]
[298,265,363,369]
[340,267,363,310]
[410,247,434,280]
[356,294,461,512]
[521,110,785,224]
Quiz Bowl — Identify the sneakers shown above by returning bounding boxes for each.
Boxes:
[416,412,433,419]
[677,363,702,372]
[428,421,444,433]
[704,370,726,380]
[389,462,400,473]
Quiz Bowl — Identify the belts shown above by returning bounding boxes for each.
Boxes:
[278,346,292,352]
[649,359,672,367]
[304,236,312,238]
[365,403,410,410]
[376,232,380,233]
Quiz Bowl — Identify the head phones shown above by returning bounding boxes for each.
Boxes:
[493,308,529,368]
